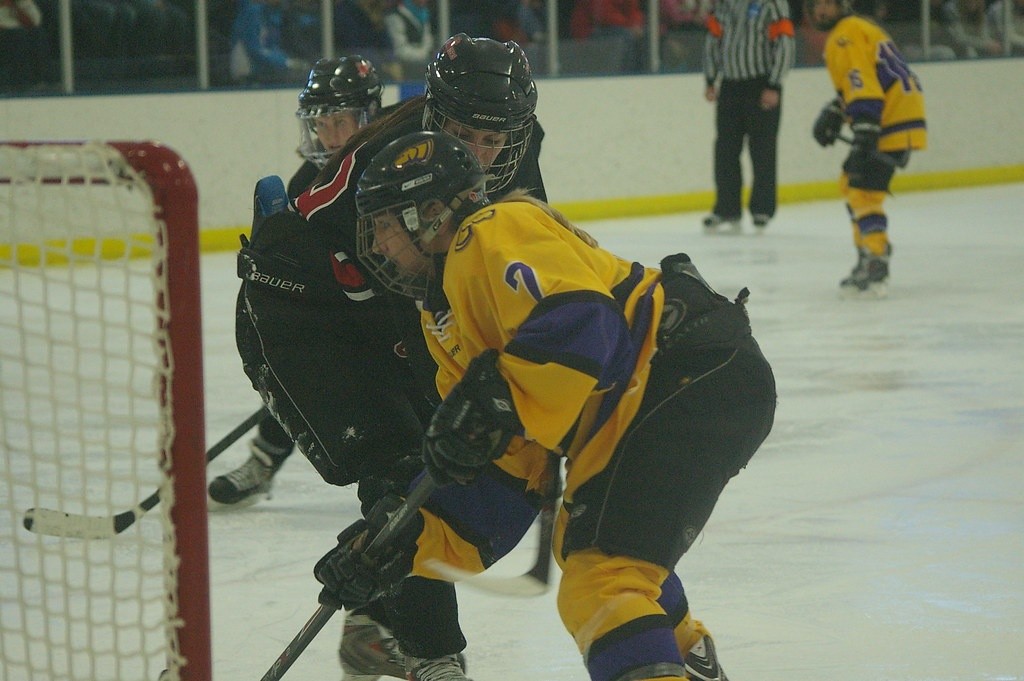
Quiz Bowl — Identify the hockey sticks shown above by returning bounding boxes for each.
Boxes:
[23,409,268,542]
[829,131,913,167]
[260,416,488,681]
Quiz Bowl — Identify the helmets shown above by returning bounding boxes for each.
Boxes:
[297,54,386,124]
[424,33,539,196]
[354,130,490,308]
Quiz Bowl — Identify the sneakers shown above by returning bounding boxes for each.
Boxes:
[683,635,729,681]
[703,213,740,230]
[839,250,892,293]
[403,655,475,681]
[751,212,771,228]
[337,614,468,681]
[207,454,277,505]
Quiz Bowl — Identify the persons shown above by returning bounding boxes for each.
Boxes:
[208,55,386,512]
[234,32,548,681]
[703,0,796,235]
[355,132,778,681]
[805,0,928,300]
[0,0,1024,99]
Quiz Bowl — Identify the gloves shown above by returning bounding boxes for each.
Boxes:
[420,356,520,492]
[312,492,426,610]
[811,100,846,147]
[845,129,880,187]
